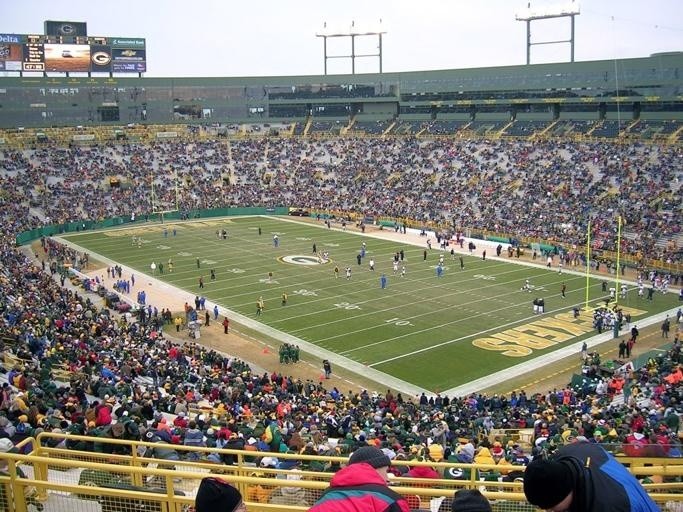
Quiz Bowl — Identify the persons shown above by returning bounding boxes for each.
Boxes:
[0,120,683,512]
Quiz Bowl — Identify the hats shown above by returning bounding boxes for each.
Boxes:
[349,446,391,468]
[195,477,242,512]
[452,488,492,512]
[524,459,572,509]
